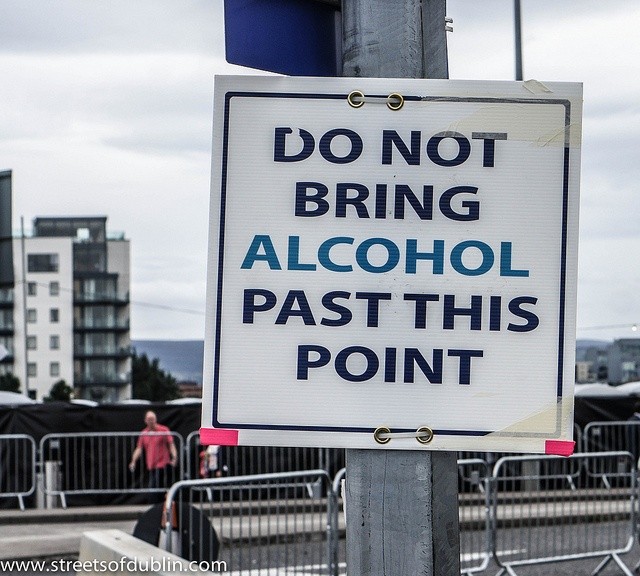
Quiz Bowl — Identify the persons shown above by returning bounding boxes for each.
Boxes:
[129,411,178,488]
[199,445,222,478]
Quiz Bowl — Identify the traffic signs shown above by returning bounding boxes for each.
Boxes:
[224,0,336,76]
